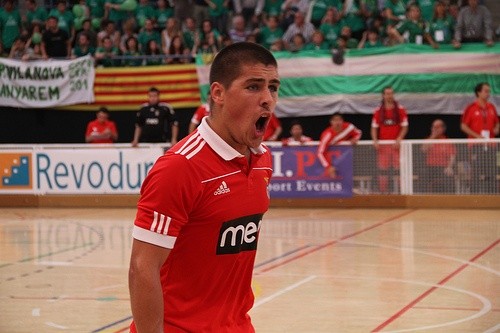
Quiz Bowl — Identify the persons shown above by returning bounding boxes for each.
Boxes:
[261,111,283,142]
[371,86,409,193]
[460,83,500,191]
[188,90,211,137]
[280,123,314,142]
[317,111,362,180]
[422,120,456,192]
[127,42,283,332]
[131,86,179,148]
[0,0,500,67]
[86,107,118,143]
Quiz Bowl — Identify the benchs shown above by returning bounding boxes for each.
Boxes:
[353,176,418,194]
